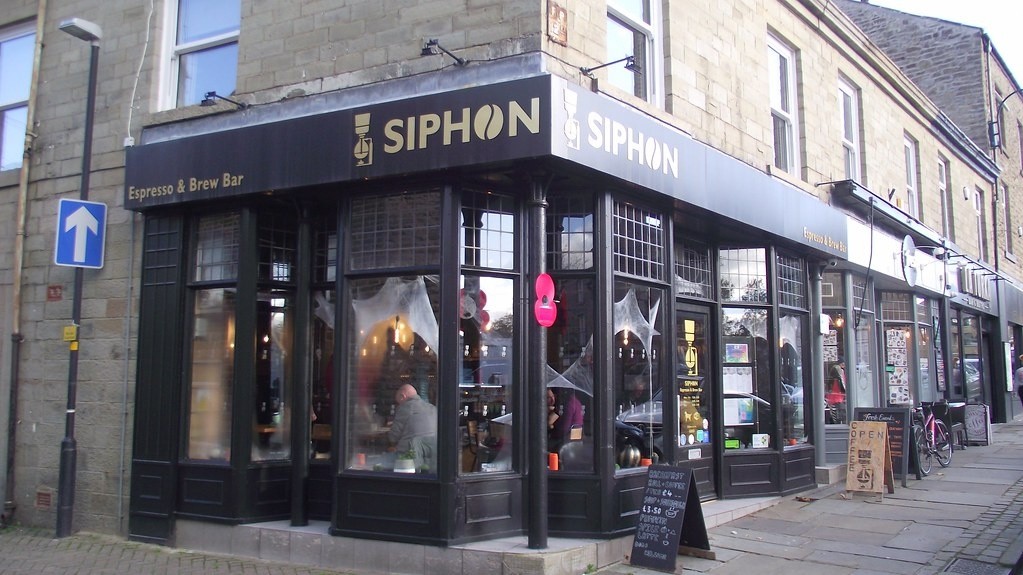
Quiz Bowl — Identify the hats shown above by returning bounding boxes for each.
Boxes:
[838,355,845,364]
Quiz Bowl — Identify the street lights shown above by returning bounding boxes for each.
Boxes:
[54,17,105,539]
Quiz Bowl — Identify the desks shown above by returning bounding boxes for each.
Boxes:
[257,423,392,443]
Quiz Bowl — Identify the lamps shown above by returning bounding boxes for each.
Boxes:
[421,37,466,68]
[580,54,644,79]
[199,89,248,111]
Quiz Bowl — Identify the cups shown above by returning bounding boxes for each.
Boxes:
[357,454,365,465]
[790,439,797,445]
[640,458,653,466]
[549,453,559,471]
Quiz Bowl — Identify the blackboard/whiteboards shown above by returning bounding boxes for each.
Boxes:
[631,466,695,572]
[853,407,912,478]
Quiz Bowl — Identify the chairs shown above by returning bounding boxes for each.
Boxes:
[467,420,487,473]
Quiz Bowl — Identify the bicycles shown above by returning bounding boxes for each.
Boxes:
[912,401,952,476]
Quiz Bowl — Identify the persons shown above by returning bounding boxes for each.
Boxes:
[387,384,438,475]
[311,390,322,458]
[1014,354,1023,407]
[546,388,583,440]
[825,357,847,404]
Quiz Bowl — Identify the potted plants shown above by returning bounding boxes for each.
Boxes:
[393,447,417,474]
